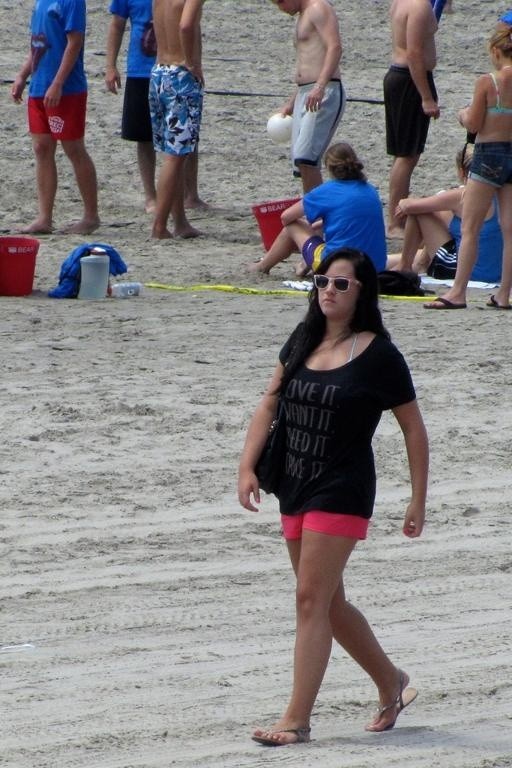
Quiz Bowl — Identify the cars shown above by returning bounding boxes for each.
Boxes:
[253,198,302,254]
[1,237,40,295]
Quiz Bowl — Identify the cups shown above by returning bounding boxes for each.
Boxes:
[313,274,364,294]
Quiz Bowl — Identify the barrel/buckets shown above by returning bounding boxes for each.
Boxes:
[364,670,419,731]
[423,297,468,309]
[251,728,314,746]
[487,295,511,309]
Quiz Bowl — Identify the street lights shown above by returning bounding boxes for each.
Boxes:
[252,405,288,496]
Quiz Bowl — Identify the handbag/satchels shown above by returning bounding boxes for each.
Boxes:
[313,82,328,92]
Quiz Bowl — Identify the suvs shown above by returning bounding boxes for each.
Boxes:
[0,235,39,298]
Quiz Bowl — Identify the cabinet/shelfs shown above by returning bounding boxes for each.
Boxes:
[106,281,141,297]
[78,247,110,299]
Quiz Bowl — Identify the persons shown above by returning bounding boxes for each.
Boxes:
[243,140,387,278]
[383,0,441,240]
[420,7,512,310]
[11,0,101,235]
[267,0,347,196]
[148,0,207,241]
[236,247,429,746]
[104,0,214,215]
[385,142,503,284]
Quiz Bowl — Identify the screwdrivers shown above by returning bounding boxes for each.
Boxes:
[266,114,295,144]
